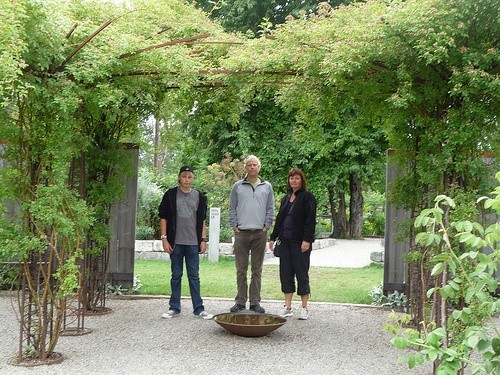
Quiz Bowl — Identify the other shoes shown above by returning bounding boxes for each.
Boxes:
[250,305,265,313]
[230,303,245,312]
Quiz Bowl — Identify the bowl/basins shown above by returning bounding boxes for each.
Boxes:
[211,313,287,338]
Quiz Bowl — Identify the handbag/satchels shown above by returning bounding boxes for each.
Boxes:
[274,240,281,257]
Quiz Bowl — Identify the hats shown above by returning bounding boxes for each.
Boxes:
[179,166,194,174]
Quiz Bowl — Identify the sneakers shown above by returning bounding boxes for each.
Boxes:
[162,309,176,318]
[298,307,309,320]
[280,307,293,318]
[200,311,214,319]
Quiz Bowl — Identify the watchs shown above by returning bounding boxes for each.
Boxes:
[161,235,167,239]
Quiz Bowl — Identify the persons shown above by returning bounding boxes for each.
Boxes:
[269,169,316,320]
[159,166,214,320]
[228,155,273,313]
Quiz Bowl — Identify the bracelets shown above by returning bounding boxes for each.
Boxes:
[202,238,208,242]
